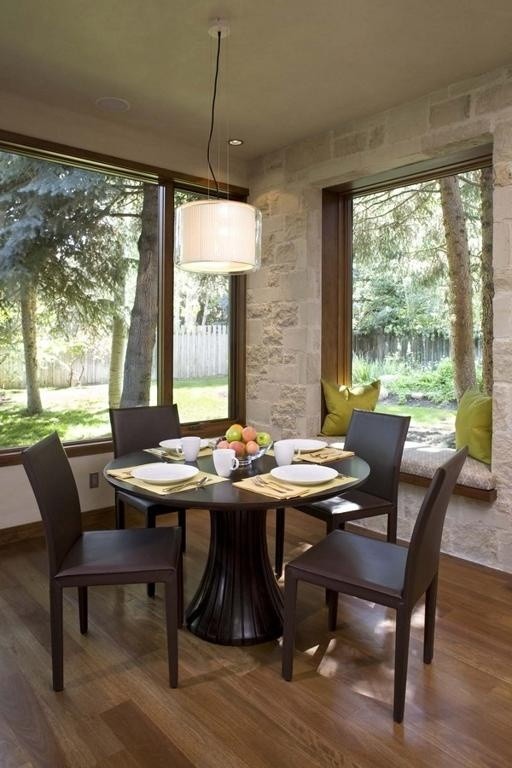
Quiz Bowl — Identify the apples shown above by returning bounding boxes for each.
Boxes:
[225,428,241,443]
[256,432,272,447]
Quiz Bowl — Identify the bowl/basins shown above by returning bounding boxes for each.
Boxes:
[207,439,272,465]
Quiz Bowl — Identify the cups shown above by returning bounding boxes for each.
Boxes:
[212,448,240,478]
[272,441,302,467]
[180,436,202,463]
[211,479,240,503]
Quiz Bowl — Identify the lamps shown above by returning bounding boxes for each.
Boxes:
[174,18,264,279]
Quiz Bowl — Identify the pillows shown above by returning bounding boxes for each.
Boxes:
[454,384,493,465]
[318,375,382,438]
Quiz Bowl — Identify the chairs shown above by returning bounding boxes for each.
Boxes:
[106,403,193,600]
[273,409,414,609]
[17,428,186,693]
[281,444,476,726]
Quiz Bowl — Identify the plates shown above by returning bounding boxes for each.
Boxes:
[129,462,199,485]
[270,463,338,486]
[158,438,210,454]
[274,438,329,454]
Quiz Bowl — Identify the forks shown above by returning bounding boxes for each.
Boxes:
[161,475,208,492]
[252,476,294,494]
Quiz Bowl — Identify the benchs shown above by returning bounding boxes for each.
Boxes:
[320,432,497,503]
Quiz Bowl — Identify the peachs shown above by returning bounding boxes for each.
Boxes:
[216,440,229,449]
[228,441,245,455]
[242,427,257,442]
[245,441,259,453]
